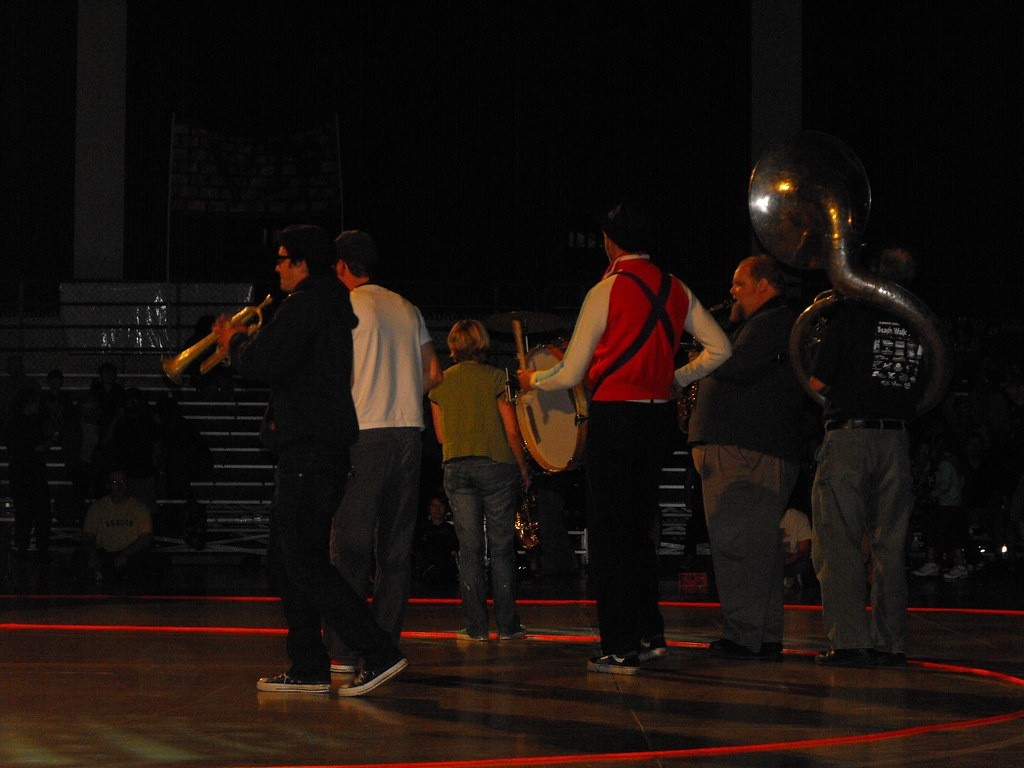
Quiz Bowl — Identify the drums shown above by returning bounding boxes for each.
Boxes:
[516,342,592,475]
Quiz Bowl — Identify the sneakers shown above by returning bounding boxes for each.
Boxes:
[338,653,409,696]
[639,635,668,661]
[255,668,331,693]
[587,653,640,675]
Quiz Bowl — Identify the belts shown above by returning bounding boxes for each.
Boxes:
[826,419,906,430]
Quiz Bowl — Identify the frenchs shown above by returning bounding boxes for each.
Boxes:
[748,132,956,416]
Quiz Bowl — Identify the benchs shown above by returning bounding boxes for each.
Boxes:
[0,280,691,558]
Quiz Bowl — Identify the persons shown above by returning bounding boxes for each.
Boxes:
[213,224,410,697]
[517,201,733,676]
[427,320,531,641]
[412,497,462,598]
[809,232,927,666]
[0,358,207,601]
[325,230,443,674]
[687,255,805,663]
[778,509,820,604]
[910,384,1024,607]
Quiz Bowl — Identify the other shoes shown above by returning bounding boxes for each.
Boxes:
[499,624,527,640]
[457,628,488,640]
[814,648,872,667]
[329,655,354,673]
[870,652,906,665]
[711,638,782,658]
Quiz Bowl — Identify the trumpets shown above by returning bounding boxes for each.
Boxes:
[160,289,284,385]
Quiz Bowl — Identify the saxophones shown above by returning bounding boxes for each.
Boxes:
[676,297,737,435]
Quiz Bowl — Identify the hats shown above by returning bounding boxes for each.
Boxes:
[602,200,658,252]
[334,230,375,267]
[280,224,341,269]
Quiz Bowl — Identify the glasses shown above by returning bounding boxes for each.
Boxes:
[274,255,291,265]
